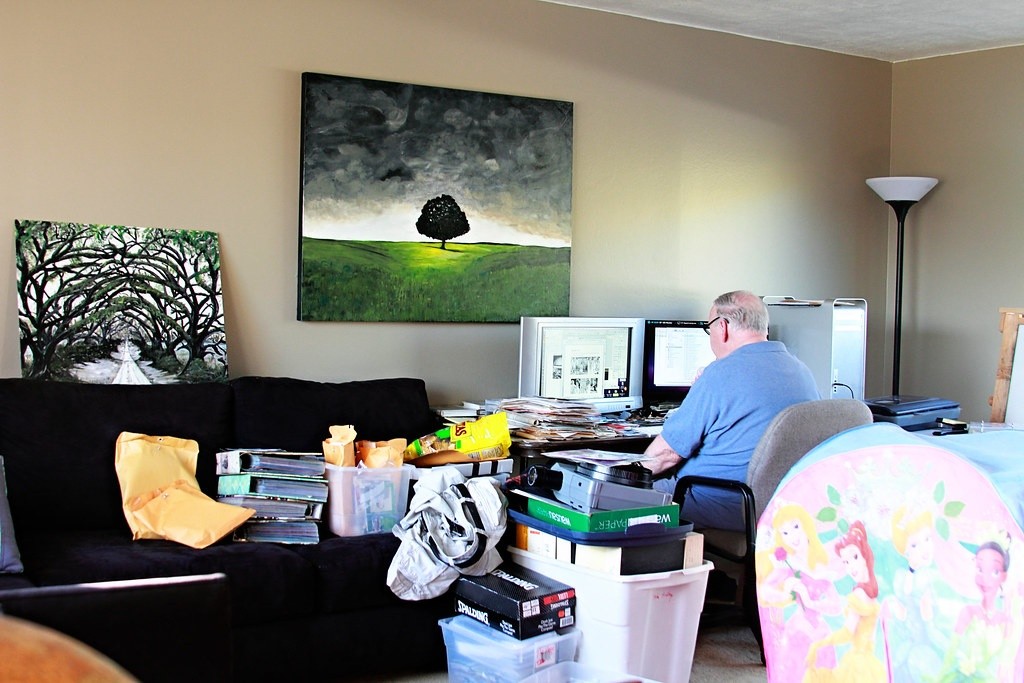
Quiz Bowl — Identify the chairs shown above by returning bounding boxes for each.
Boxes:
[675,399,873,667]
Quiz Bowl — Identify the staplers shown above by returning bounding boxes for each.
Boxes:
[932,416,969,436]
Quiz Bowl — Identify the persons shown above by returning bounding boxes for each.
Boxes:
[638,292,822,532]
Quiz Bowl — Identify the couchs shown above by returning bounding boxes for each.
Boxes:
[0,377,517,683]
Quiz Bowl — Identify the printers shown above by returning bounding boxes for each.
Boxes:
[865,395,961,433]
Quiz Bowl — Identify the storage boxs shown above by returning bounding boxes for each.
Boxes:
[438,486,714,683]
[323,462,415,539]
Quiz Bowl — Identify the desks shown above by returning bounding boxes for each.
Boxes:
[429,397,662,479]
[908,423,1024,472]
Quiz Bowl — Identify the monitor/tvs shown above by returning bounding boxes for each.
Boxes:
[519,316,645,414]
[643,320,717,418]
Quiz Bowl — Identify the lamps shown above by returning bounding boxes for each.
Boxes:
[864,176,940,397]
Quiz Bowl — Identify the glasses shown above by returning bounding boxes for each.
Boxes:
[702,316,729,335]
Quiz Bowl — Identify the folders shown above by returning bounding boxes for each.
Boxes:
[212,446,332,545]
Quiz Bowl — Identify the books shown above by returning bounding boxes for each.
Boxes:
[216,451,329,544]
[429,400,485,424]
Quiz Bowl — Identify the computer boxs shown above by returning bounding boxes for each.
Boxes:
[759,295,868,402]
